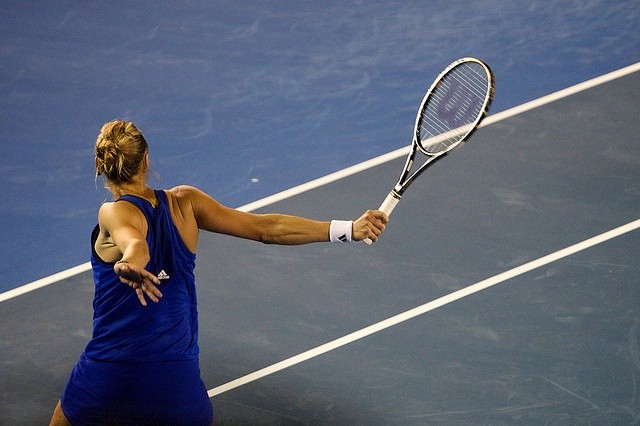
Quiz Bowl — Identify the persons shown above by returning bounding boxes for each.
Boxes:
[49,119,388,425]
